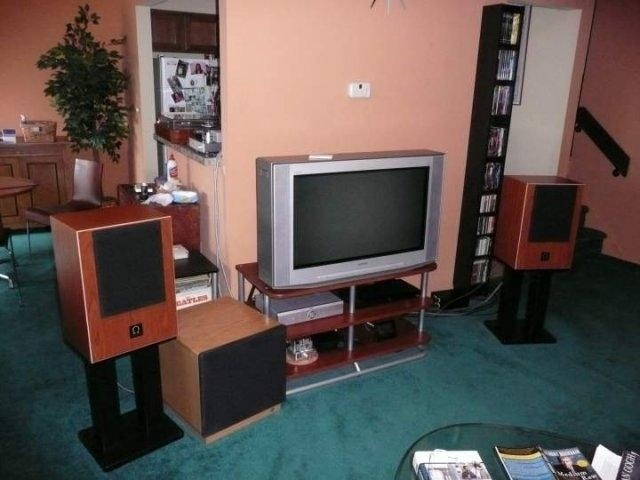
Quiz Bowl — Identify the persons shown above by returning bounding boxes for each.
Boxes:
[462,465,477,478]
[476,463,489,479]
[554,454,590,480]
[193,63,205,74]
[206,68,216,86]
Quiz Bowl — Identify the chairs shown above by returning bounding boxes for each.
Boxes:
[24,157,104,257]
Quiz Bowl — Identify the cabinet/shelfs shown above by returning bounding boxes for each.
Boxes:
[235,262,437,396]
[150,9,217,54]
[454,4,525,307]
[0,136,95,230]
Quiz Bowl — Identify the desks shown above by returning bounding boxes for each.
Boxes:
[393,423,594,480]
[0,175,39,288]
[174,253,219,311]
[118,183,201,253]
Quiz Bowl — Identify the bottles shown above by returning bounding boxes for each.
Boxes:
[167,153,178,182]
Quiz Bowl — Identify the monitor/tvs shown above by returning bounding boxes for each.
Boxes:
[254,148,445,291]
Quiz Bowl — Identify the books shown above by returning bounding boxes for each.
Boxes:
[411,450,493,480]
[494,443,601,480]
[615,448,640,480]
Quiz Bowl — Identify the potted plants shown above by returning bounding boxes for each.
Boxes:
[36,1,132,209]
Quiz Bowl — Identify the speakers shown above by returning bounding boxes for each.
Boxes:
[50,204,184,472]
[483,175,585,344]
[159,296,287,445]
